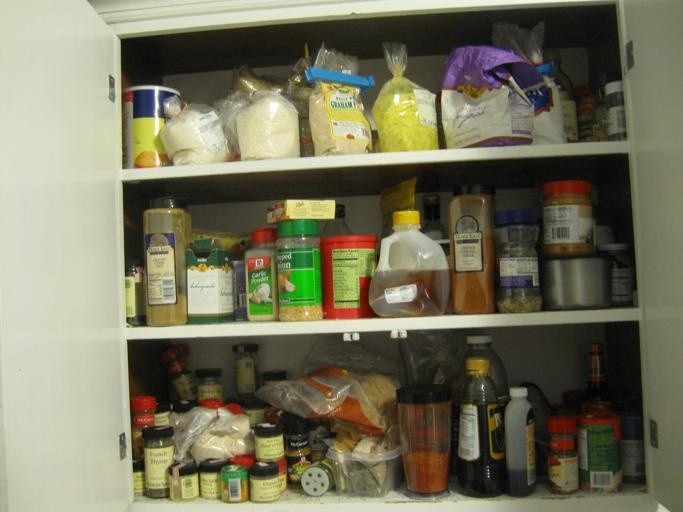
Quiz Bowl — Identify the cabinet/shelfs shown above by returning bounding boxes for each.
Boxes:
[145,426,175,496]
[603,243,634,306]
[254,422,283,462]
[448,178,497,313]
[133,459,145,498]
[575,83,598,136]
[505,386,536,496]
[275,461,289,494]
[143,194,191,316]
[578,343,625,493]
[456,359,504,498]
[495,208,541,312]
[421,194,443,238]
[168,361,195,404]
[126,257,144,327]
[251,462,281,502]
[542,180,595,257]
[606,80,625,138]
[302,461,333,496]
[286,435,309,486]
[130,397,155,453]
[233,343,258,398]
[199,460,223,499]
[459,335,506,403]
[324,203,351,239]
[245,227,278,321]
[221,466,247,501]
[196,366,222,409]
[170,464,198,500]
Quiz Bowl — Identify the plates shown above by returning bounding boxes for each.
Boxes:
[0,0,682,510]
